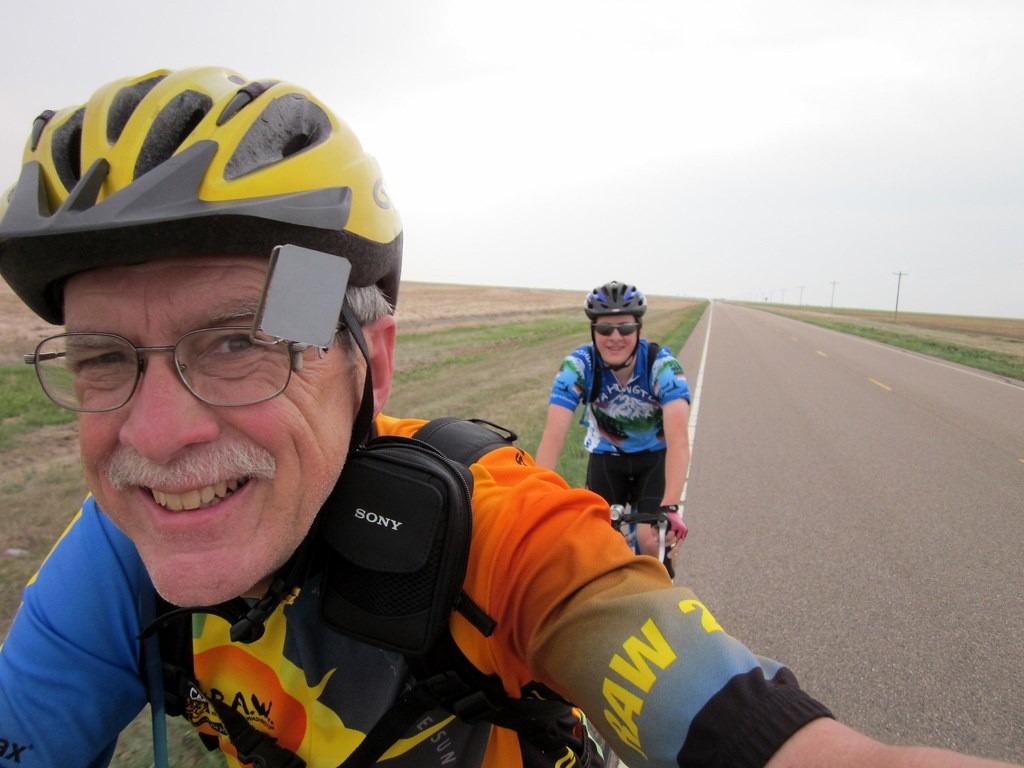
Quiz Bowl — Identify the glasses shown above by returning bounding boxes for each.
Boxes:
[23,326,333,413]
[592,323,641,336]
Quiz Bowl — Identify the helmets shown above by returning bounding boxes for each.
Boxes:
[583,280,647,320]
[0,65,402,313]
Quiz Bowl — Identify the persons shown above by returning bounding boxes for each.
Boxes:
[535,277,692,580]
[0,63,1024,768]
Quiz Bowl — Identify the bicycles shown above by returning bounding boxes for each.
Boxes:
[605,475,681,580]
